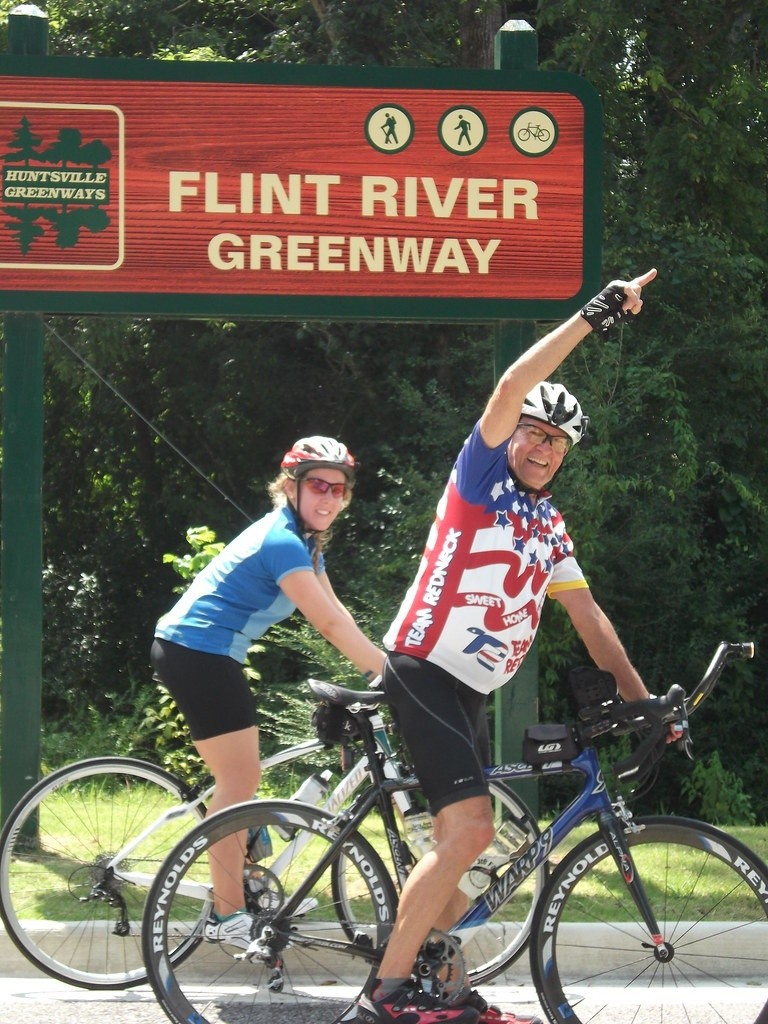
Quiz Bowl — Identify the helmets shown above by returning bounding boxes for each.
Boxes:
[520,381,591,445]
[280,436,357,490]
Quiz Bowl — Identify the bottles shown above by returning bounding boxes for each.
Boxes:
[245,795,272,863]
[402,799,437,862]
[456,814,531,900]
[270,770,332,841]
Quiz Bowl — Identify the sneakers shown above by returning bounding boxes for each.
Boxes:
[258,891,318,919]
[202,911,293,952]
[357,978,480,1024]
[424,989,544,1024]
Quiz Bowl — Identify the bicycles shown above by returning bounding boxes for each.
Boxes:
[142,640,768,1024]
[0,662,550,992]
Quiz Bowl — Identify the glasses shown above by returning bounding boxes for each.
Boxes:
[517,422,571,457]
[302,477,346,499]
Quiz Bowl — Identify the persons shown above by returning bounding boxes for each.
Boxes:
[152,436,385,950]
[360,267,685,1024]
